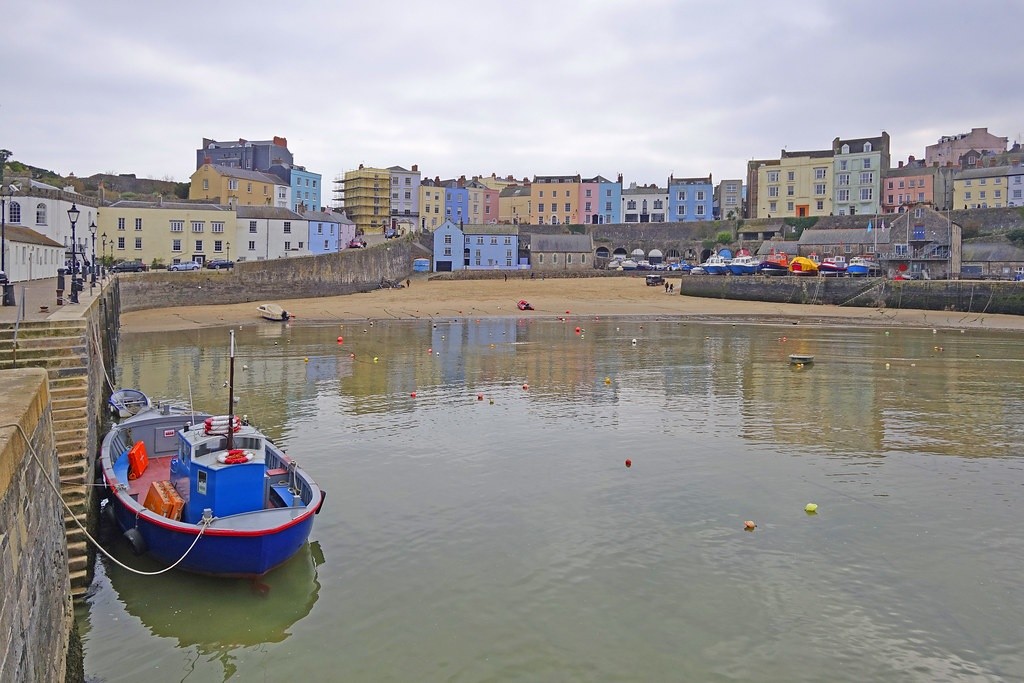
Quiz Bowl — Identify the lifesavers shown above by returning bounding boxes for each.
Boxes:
[204,414,241,434]
[216,450,255,464]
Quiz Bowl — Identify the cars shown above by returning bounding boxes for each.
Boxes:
[385,229,400,239]
[207,258,235,270]
[108,261,147,273]
[348,238,367,248]
[166,261,200,272]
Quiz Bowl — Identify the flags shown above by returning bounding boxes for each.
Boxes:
[881,220,885,231]
[866,220,872,233]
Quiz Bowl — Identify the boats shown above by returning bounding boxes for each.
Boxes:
[759,247,791,276]
[256,304,289,321]
[846,256,881,278]
[788,354,815,364]
[517,300,534,311]
[597,251,707,276]
[726,247,764,277]
[96,328,327,582]
[817,256,849,277]
[789,252,823,276]
[700,248,732,276]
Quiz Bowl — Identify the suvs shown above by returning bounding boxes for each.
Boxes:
[646,275,665,286]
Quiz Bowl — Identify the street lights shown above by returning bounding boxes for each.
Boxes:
[89,220,97,288]
[0,183,21,284]
[67,202,81,304]
[101,232,107,279]
[109,240,114,274]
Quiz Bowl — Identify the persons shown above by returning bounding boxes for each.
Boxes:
[406,278,411,287]
[363,241,367,248]
[665,282,668,292]
[84,259,90,273]
[66,260,72,273]
[75,260,80,273]
[669,283,674,293]
[504,273,507,282]
